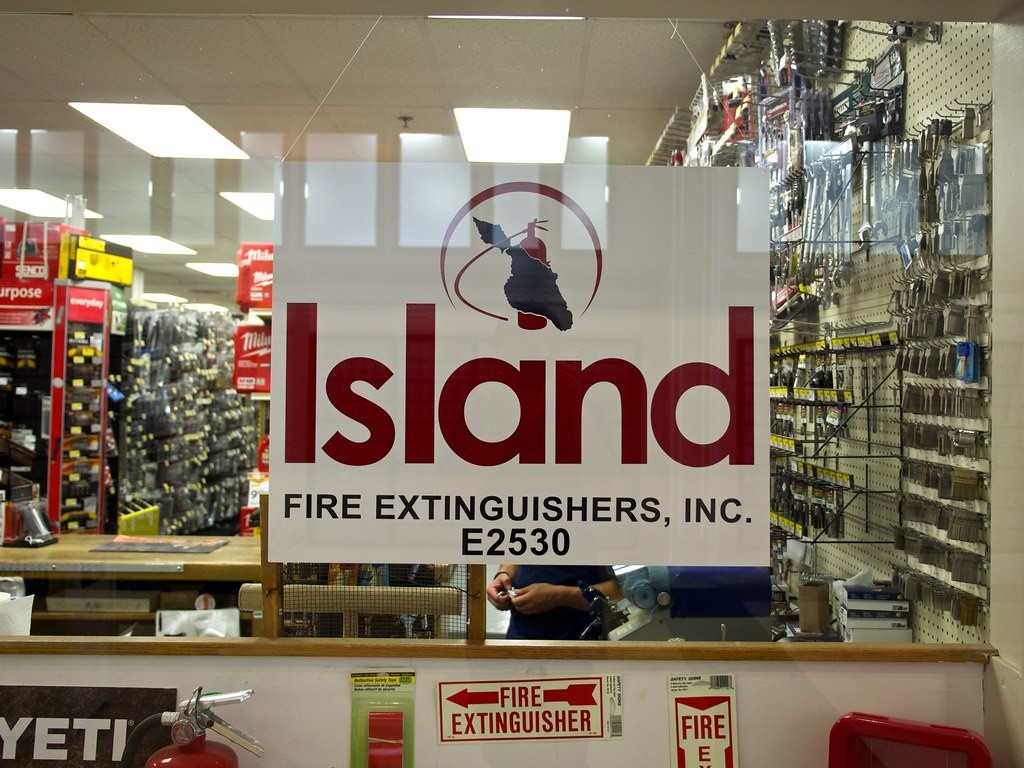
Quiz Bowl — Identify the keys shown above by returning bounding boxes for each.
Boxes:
[796,571,835,591]
[898,102,988,260]
[889,266,982,628]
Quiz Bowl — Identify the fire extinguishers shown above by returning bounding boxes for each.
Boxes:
[117,686,265,768]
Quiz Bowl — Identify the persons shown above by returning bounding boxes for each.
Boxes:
[487,564,625,642]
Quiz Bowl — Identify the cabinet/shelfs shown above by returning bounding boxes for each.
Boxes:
[0,534,262,637]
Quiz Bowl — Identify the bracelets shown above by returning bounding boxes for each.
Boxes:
[492,571,510,580]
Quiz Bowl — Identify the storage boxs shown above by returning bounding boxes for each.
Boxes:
[4,221,89,280]
[237,241,274,310]
[231,326,271,393]
[58,235,132,287]
